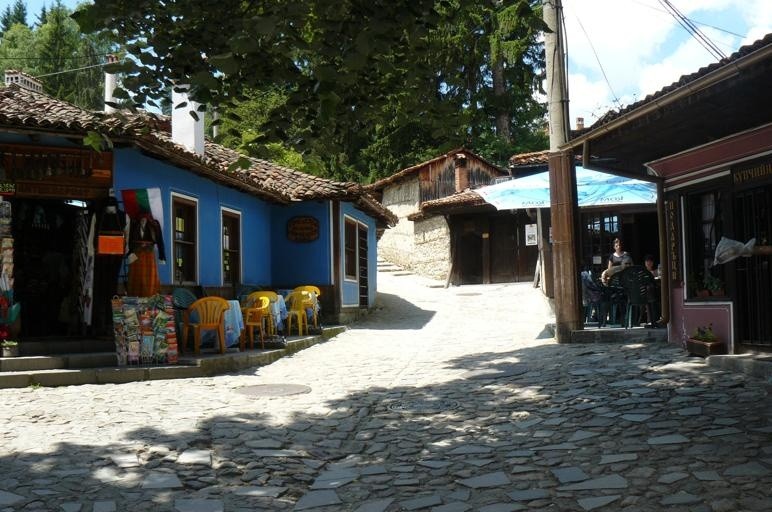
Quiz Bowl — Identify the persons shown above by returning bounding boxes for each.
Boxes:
[608,237,634,268]
[86,206,131,339]
[126,216,167,296]
[639,255,658,277]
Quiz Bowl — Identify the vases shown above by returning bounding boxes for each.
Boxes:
[686,336,728,358]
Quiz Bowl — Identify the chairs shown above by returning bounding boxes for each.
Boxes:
[170,284,322,354]
[581,270,657,328]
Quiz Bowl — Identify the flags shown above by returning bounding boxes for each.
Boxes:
[122,186,164,235]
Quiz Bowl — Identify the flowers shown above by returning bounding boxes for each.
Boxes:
[694,324,715,341]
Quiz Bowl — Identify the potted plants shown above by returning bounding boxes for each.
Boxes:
[690,274,724,300]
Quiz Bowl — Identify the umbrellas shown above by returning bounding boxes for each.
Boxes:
[473,165,659,212]
